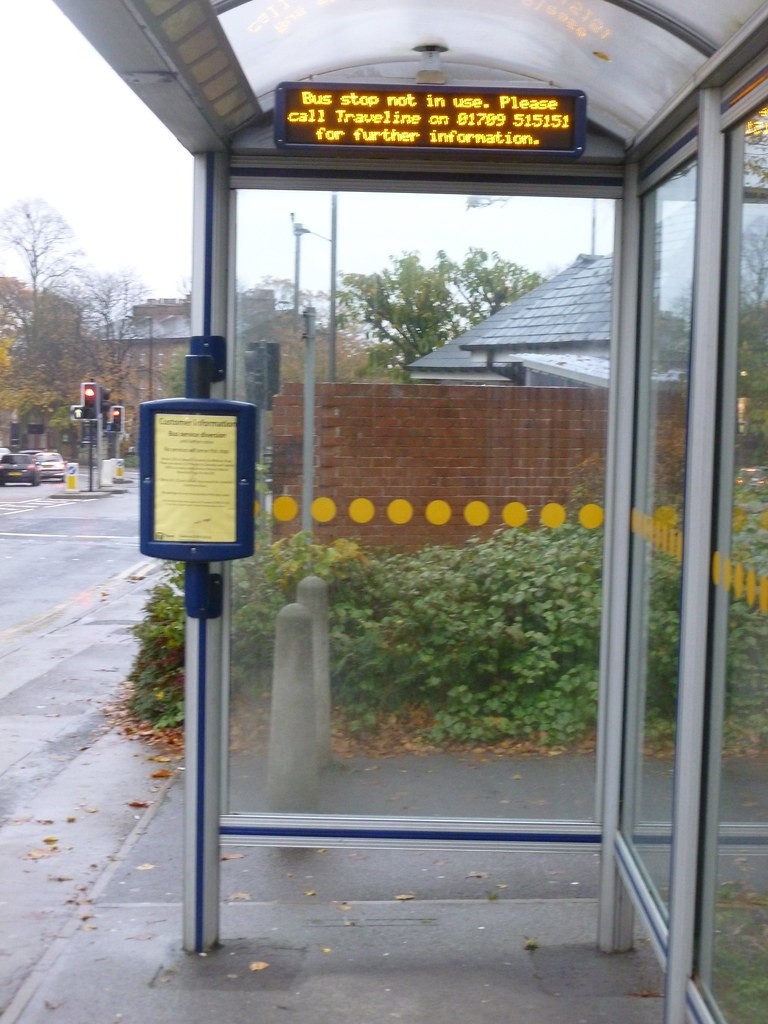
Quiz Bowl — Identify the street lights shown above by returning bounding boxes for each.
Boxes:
[294,226,337,383]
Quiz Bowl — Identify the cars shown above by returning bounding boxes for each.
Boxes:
[0,447,11,461]
[33,452,66,482]
[0,453,41,486]
[18,450,45,458]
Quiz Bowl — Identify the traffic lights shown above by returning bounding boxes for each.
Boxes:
[99,385,114,412]
[81,383,100,422]
[69,405,84,422]
[108,406,125,433]
